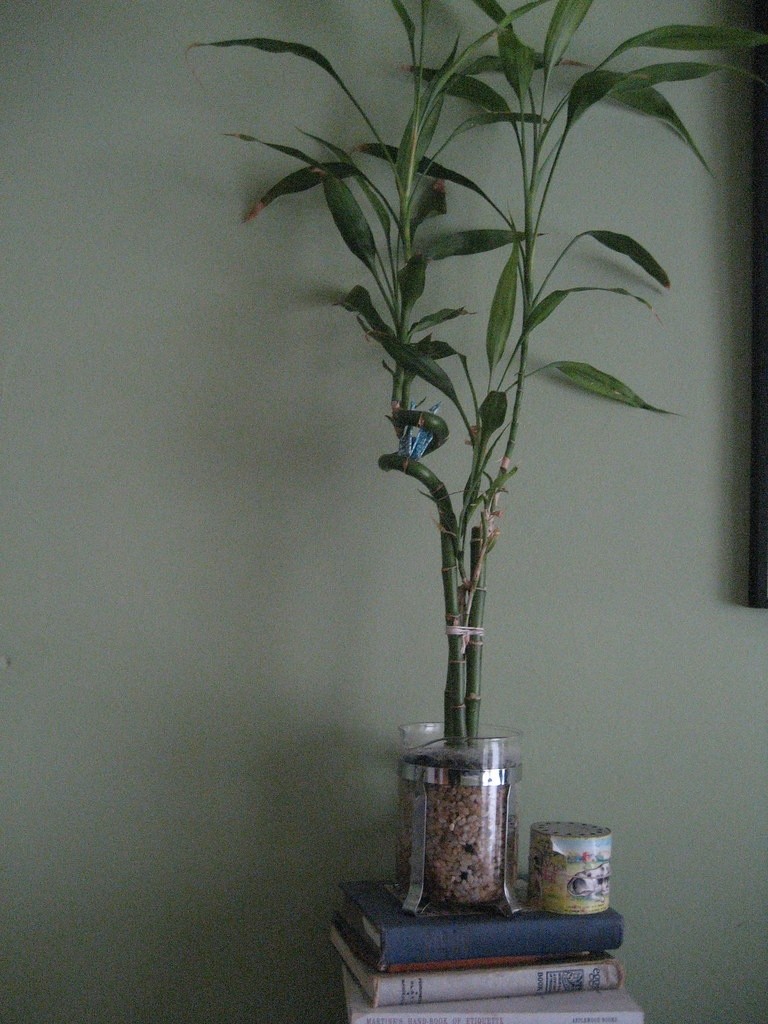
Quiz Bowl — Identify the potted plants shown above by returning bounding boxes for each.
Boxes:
[182,0,768,921]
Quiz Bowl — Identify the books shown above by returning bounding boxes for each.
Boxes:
[327,870,644,1024]
